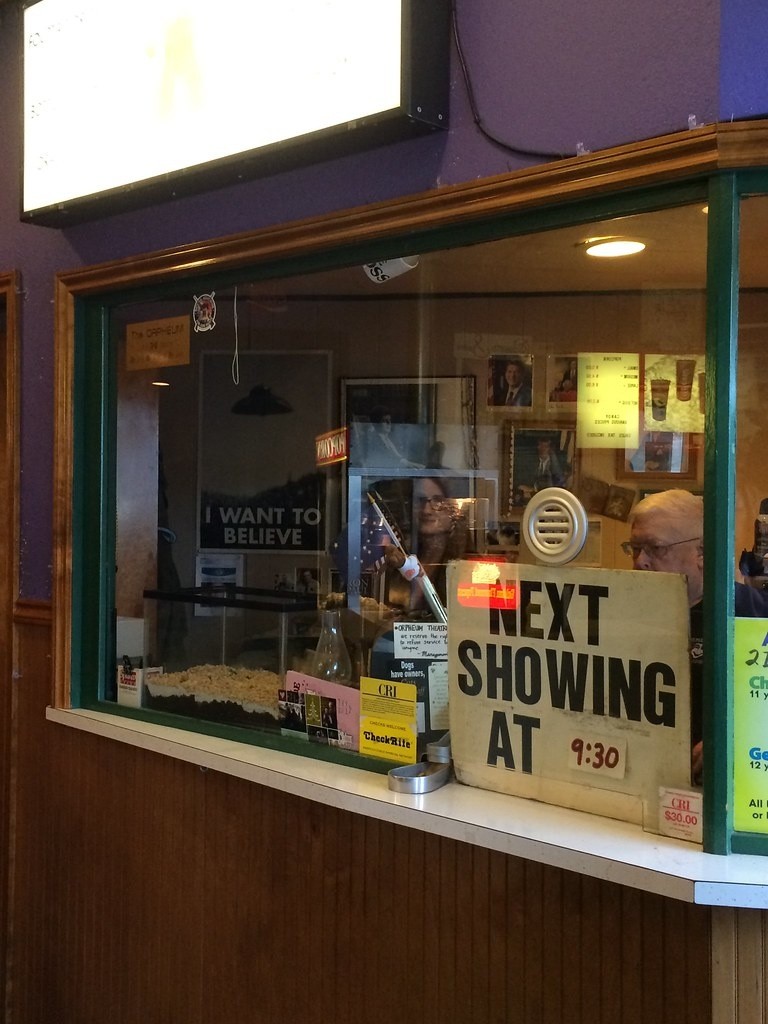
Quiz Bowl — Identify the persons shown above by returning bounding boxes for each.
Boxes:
[621,487,768,780]
[493,362,531,406]
[362,405,426,468]
[406,463,481,618]
[518,436,567,500]
[553,359,577,393]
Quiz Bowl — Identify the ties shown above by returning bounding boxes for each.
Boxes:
[506,392,514,405]
[541,461,543,473]
[572,370,574,376]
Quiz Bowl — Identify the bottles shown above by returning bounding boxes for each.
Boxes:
[314,609,350,689]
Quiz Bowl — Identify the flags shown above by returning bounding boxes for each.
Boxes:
[329,503,397,587]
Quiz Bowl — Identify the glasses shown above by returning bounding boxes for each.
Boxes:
[418,495,447,511]
[621,538,700,561]
